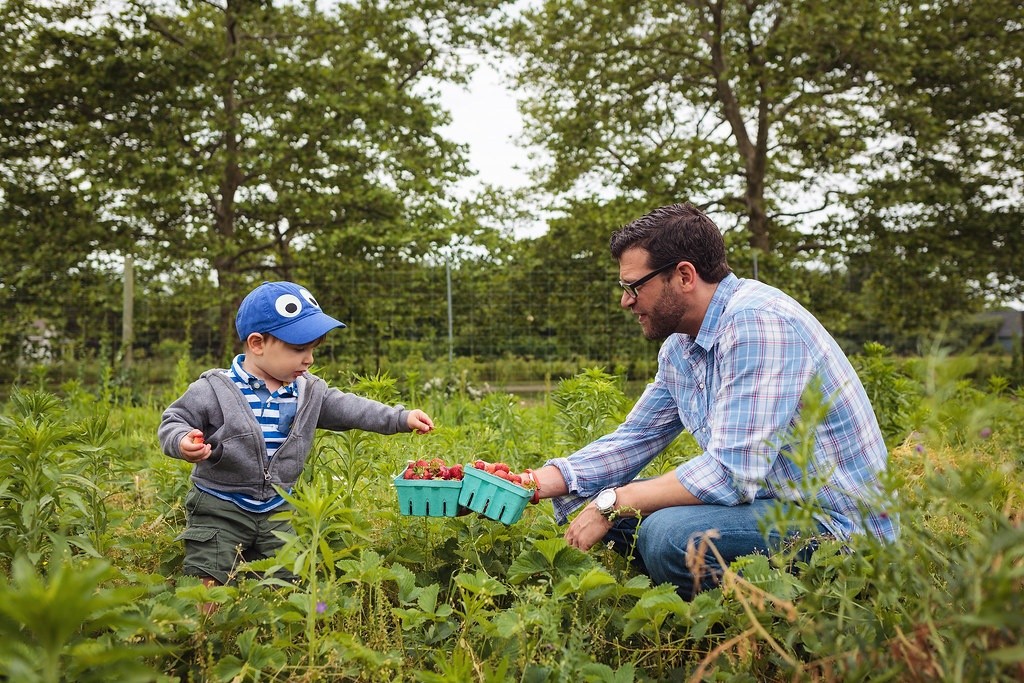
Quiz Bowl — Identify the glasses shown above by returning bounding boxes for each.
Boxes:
[618,259,678,297]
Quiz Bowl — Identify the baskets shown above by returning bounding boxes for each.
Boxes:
[394,461,534,525]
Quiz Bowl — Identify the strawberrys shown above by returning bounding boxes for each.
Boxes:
[403,459,463,481]
[472,459,529,489]
[193,436,205,444]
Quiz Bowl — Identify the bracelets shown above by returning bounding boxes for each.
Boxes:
[523,468,540,505]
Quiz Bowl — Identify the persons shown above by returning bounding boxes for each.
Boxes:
[516,202,901,633]
[157,280,435,616]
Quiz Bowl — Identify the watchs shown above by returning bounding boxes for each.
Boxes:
[594,487,621,521]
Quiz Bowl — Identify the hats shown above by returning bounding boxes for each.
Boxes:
[235,280,347,344]
